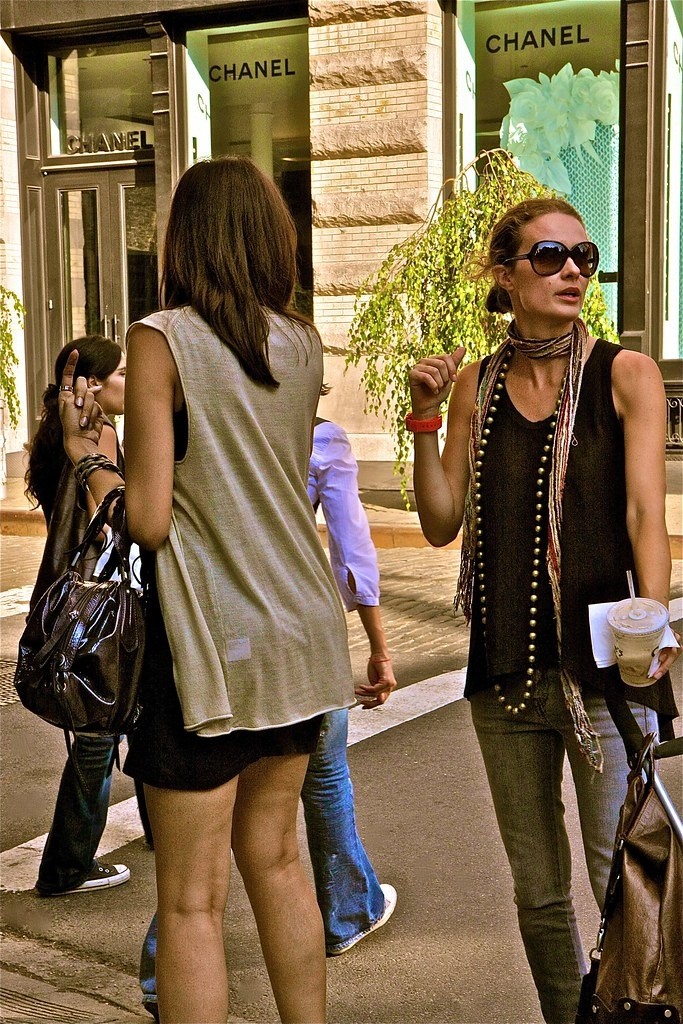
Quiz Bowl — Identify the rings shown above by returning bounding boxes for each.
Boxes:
[59,386,74,392]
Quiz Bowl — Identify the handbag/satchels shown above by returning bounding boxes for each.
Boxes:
[574,733,683,1024]
[14,487,150,737]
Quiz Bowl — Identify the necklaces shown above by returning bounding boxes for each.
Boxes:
[475,345,569,716]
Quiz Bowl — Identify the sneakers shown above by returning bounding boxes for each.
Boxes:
[38,864,130,896]
[144,1002,160,1022]
[326,884,397,954]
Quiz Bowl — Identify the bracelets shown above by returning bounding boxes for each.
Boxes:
[369,658,390,664]
[75,453,125,492]
[406,412,443,433]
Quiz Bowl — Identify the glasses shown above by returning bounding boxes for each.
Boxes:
[502,240,599,279]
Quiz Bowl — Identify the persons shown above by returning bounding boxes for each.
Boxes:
[139,381,396,1024]
[59,156,357,1024]
[406,199,683,1024]
[23,335,155,897]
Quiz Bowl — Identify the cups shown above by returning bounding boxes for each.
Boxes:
[607,597,670,688]
